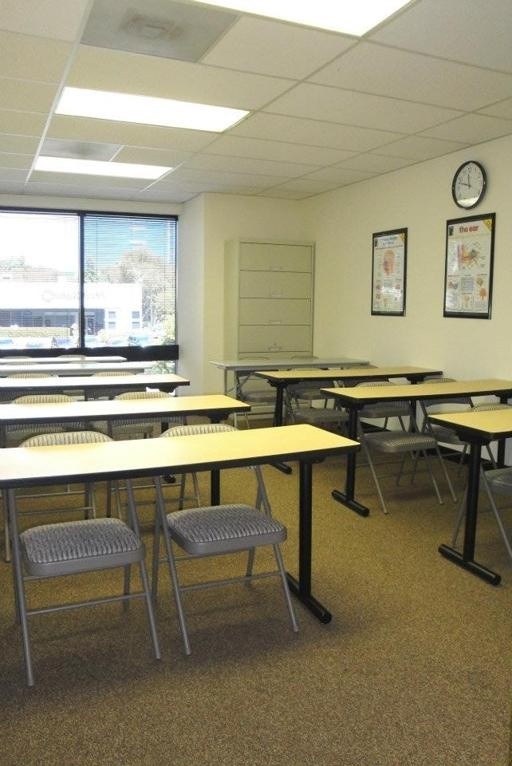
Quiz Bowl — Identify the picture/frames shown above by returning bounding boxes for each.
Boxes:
[441,213,497,319]
[370,227,409,317]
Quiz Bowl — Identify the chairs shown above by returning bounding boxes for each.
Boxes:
[0,353,251,535]
[5,431,161,687]
[453,405,512,564]
[152,423,300,653]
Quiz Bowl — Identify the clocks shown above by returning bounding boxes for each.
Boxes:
[451,160,487,212]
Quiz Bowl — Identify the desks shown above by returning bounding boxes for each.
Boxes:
[426,407,512,587]
[318,378,512,516]
[0,422,361,625]
[208,356,497,513]
